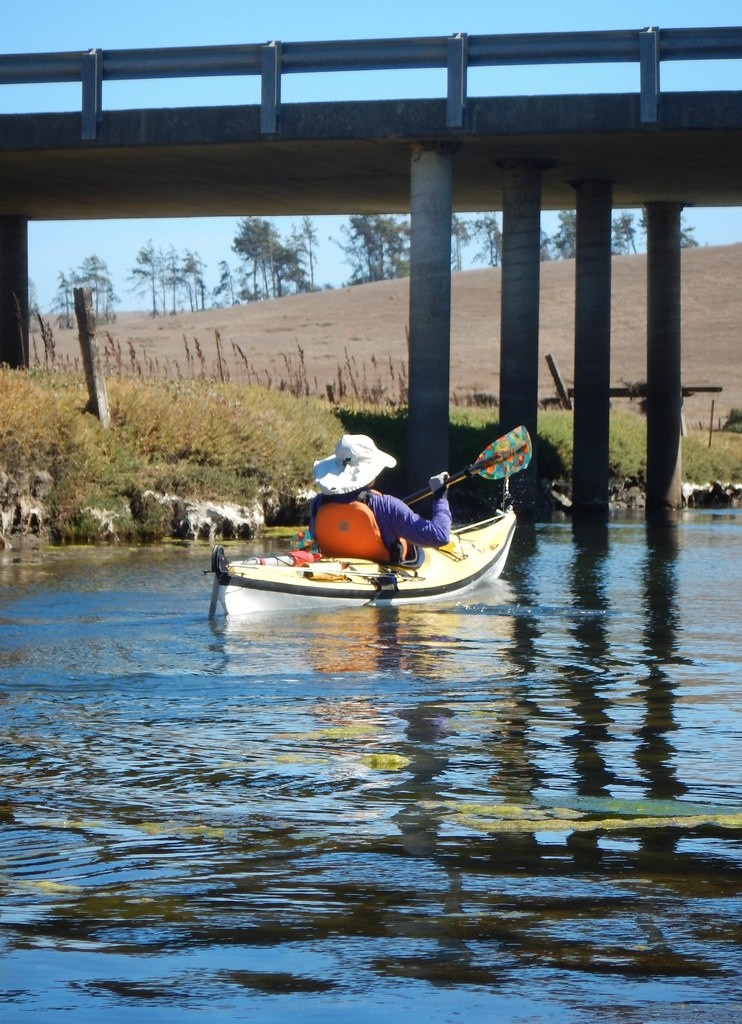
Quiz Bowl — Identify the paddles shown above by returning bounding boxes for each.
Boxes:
[287,426,533,551]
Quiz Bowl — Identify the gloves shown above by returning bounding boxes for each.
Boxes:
[429,471,451,499]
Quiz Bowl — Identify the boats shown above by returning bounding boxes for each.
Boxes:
[209,506,518,615]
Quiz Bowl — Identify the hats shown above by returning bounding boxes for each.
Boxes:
[313,434,397,496]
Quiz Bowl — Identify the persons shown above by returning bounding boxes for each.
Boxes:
[309,434,452,563]
